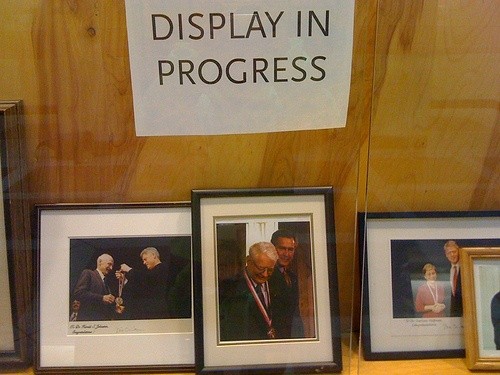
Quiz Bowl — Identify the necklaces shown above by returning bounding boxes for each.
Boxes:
[222,242,279,339]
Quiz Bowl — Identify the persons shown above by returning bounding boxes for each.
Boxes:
[443,239,464,317]
[415,262,448,317]
[76,253,125,321]
[115,247,181,319]
[267,229,305,338]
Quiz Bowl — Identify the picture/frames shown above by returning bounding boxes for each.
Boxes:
[191,186,345,375]
[356,211,500,363]
[458,247,500,372]
[32,200,197,375]
[0,99,34,372]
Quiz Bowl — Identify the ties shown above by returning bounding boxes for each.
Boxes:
[283,270,291,288]
[103,277,108,290]
[257,284,265,308]
[453,265,456,294]
